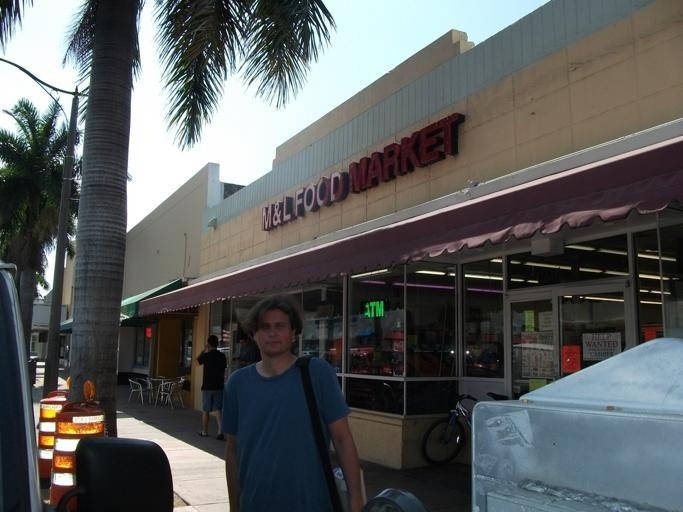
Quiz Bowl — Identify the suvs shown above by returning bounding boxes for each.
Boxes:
[0,258,173,512]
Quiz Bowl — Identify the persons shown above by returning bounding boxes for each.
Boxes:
[197,335,227,440]
[221,293,363,511]
[237,332,257,368]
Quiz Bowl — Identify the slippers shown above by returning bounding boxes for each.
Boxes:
[198,431,208,437]
[215,432,224,440]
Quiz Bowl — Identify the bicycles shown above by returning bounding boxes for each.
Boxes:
[420,390,508,465]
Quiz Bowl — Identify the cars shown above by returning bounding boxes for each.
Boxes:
[447,344,472,360]
[467,345,484,357]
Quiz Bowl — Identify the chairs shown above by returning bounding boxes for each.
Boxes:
[55,438,174,512]
[127,375,188,409]
